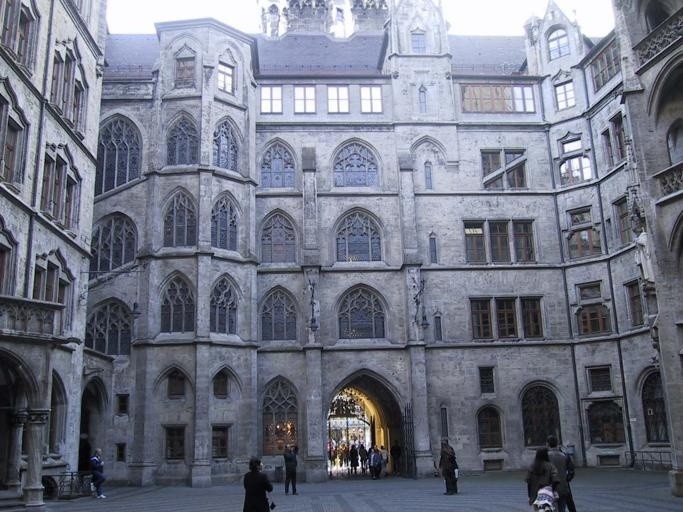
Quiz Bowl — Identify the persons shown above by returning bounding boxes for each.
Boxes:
[282,443,302,495]
[243,457,273,511]
[327,437,390,480]
[440,440,456,495]
[545,435,578,512]
[90,447,108,500]
[524,446,561,512]
[632,225,655,284]
[437,437,460,494]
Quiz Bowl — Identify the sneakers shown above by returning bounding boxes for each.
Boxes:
[90,483,95,491]
[97,494,106,499]
[285,492,299,495]
[444,490,456,495]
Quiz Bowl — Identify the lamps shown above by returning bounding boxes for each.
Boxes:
[400,253,430,330]
[299,262,323,332]
